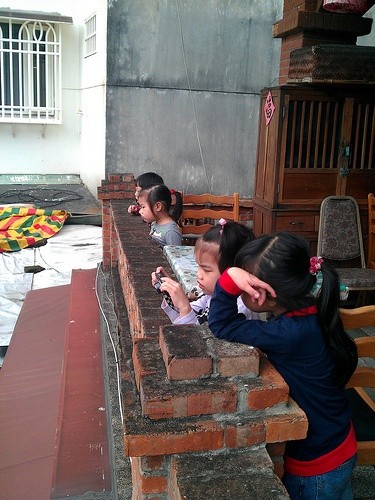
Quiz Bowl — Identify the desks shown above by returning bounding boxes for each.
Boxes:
[162,244,349,321]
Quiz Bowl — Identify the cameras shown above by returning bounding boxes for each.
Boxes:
[154,272,169,297]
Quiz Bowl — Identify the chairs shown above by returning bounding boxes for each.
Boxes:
[366,193,375,305]
[339,305,375,466]
[316,195,375,308]
[178,191,239,234]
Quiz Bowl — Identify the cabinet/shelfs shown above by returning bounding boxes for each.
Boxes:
[252,85,375,306]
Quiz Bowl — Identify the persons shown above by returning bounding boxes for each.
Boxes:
[151,218,261,326]
[128,172,164,214]
[138,184,183,246]
[207,232,358,500]
